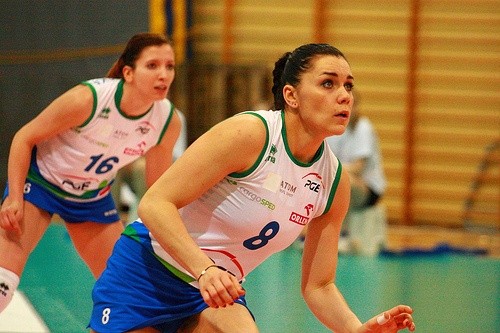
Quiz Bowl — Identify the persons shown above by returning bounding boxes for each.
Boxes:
[86,43,417,333]
[0,33,180,312]
[302,88,385,252]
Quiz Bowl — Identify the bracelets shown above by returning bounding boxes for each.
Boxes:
[199,266,215,280]
